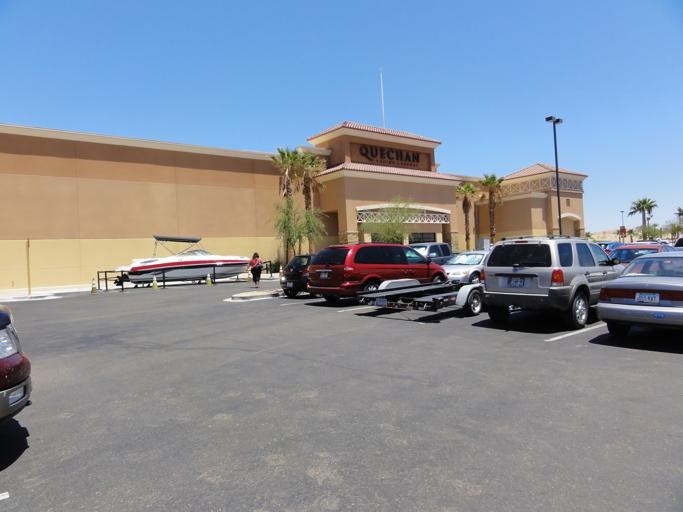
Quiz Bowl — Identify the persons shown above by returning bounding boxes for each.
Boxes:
[246,252,263,288]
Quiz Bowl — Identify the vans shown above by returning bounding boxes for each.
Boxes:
[480,236,626,329]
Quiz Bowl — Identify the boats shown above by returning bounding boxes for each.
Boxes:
[113,250,251,286]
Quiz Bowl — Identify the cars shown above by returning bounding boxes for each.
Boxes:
[279,254,323,299]
[612,245,676,273]
[627,242,669,244]
[439,250,491,285]
[0,304,32,422]
[402,242,452,266]
[594,242,627,259]
[306,243,448,305]
[672,237,682,251]
[596,250,683,338]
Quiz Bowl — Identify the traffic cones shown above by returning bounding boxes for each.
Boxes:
[204,274,213,287]
[245,269,253,284]
[89,277,98,295]
[150,275,160,290]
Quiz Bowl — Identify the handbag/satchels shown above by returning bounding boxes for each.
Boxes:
[257,265,263,270]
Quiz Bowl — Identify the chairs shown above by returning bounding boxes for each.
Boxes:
[657,269,673,275]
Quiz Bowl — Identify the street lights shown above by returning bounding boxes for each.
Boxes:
[620,210,624,245]
[545,115,563,236]
